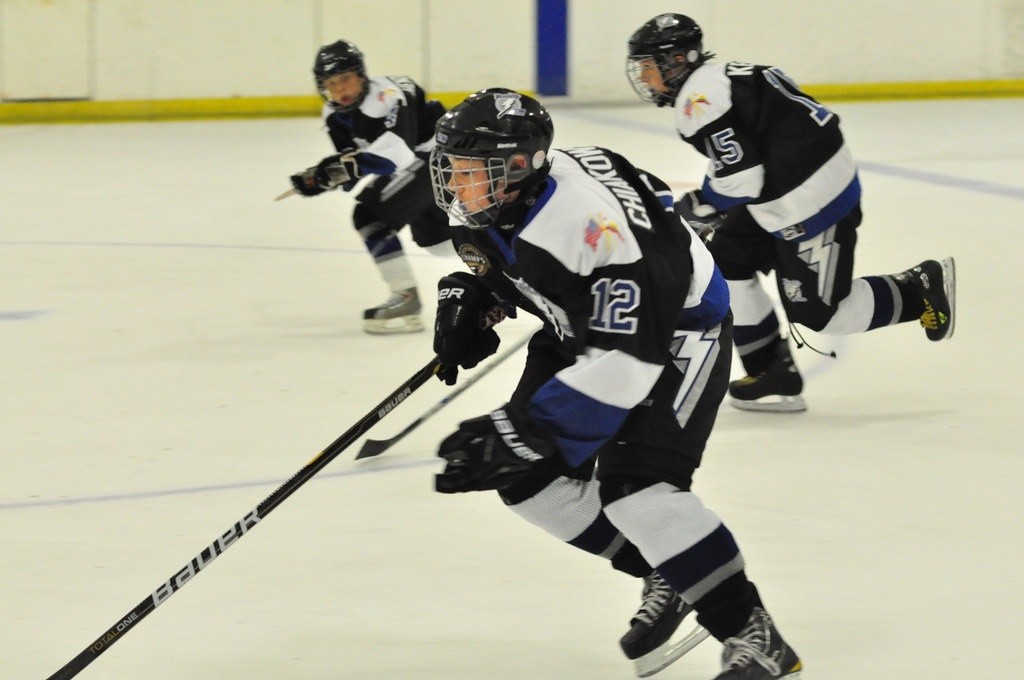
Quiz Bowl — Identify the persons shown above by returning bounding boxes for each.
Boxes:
[290,40,448,336]
[429,87,803,680]
[625,13,956,413]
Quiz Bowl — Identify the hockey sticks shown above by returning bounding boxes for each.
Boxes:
[44,303,509,680]
[354,324,535,460]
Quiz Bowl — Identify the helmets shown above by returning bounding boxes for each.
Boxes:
[429,87,554,228]
[628,13,702,104]
[312,39,367,111]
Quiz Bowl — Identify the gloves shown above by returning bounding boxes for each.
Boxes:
[434,271,500,385]
[435,401,557,494]
[674,190,719,224]
[291,154,350,196]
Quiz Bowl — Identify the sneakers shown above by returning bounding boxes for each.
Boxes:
[728,360,808,411]
[362,286,424,333]
[909,255,956,342]
[712,607,800,680]
[620,571,708,678]
